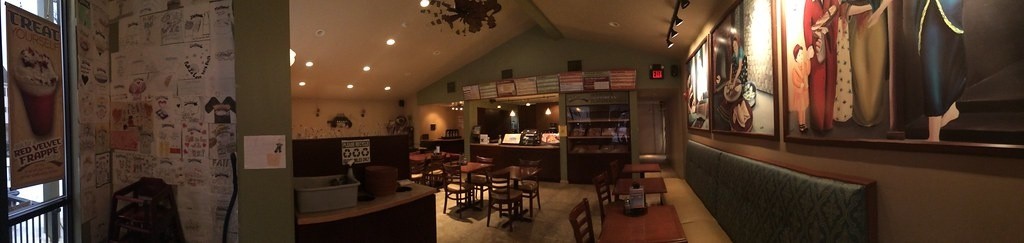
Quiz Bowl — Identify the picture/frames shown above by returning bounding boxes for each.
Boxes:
[709,0,780,140]
[778,0,1024,159]
[684,37,711,132]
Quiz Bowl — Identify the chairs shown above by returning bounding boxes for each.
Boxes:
[485,170,524,231]
[109,177,185,243]
[611,160,624,202]
[568,198,602,243]
[510,159,542,215]
[410,147,461,190]
[441,162,475,217]
[465,156,494,206]
[593,170,612,237]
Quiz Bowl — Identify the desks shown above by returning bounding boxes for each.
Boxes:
[490,166,542,228]
[449,161,494,213]
[421,138,464,150]
[622,163,660,177]
[612,177,667,204]
[409,153,448,175]
[602,203,688,243]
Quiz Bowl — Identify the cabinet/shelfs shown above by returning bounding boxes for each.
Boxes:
[295,179,441,243]
[568,117,631,154]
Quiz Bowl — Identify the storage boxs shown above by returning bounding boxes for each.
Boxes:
[294,174,360,213]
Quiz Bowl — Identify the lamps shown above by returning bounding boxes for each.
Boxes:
[675,15,683,26]
[671,29,679,38]
[667,39,674,48]
[680,0,690,9]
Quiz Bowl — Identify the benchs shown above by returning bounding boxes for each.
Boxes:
[659,134,879,243]
